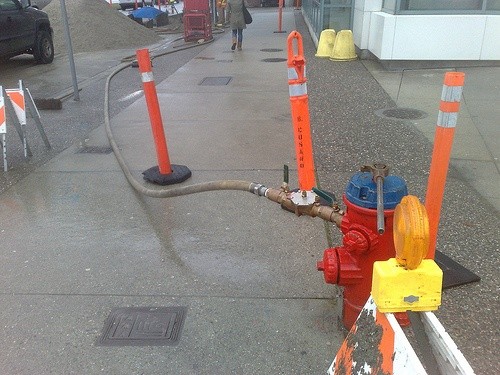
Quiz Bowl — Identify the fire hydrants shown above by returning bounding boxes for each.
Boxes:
[317,162,410,332]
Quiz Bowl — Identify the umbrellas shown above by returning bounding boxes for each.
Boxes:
[131,6,162,19]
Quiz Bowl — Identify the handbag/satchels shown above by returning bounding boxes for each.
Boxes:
[243,6,252,24]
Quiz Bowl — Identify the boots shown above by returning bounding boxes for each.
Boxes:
[237,41,241,50]
[231,37,237,50]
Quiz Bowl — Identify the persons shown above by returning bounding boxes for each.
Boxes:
[225,0,246,51]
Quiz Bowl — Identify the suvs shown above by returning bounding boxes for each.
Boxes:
[0,0,54,64]
